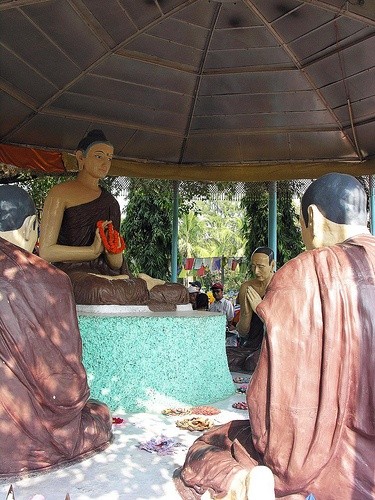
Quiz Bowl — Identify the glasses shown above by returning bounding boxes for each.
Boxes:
[212,290,220,293]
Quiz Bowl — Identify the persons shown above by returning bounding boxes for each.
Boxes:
[39,130,188,312]
[186,280,241,337]
[0,186,114,480]
[226,247,276,374]
[174,174,375,500]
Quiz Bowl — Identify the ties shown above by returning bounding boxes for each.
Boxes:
[211,283,223,289]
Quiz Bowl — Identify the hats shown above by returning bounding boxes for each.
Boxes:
[189,281,201,289]
[188,286,200,293]
